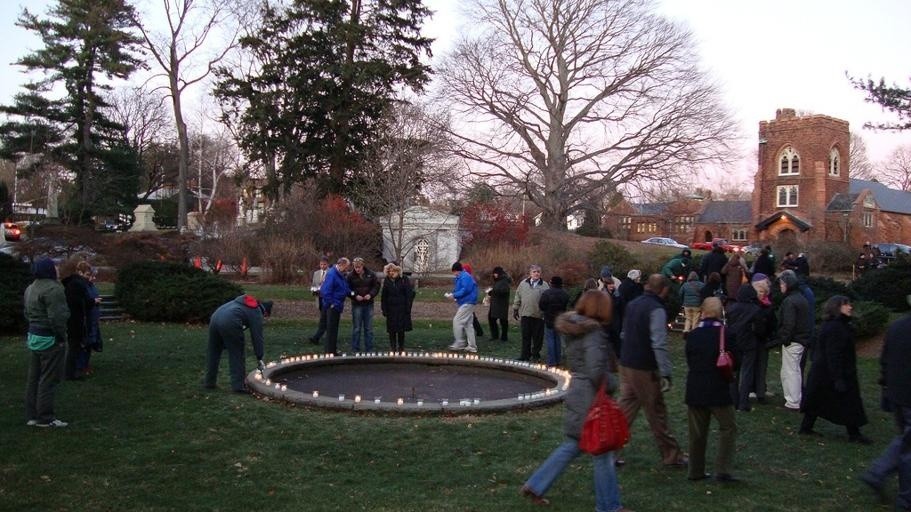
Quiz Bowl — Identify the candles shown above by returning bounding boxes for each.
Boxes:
[253,351,572,408]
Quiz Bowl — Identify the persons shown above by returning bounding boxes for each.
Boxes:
[202,293,274,394]
[22,256,69,428]
[309,256,331,345]
[86,268,102,377]
[54,260,103,381]
[346,257,381,355]
[445,244,910,512]
[319,257,356,356]
[381,261,416,353]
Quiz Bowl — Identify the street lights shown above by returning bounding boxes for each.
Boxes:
[668,219,673,238]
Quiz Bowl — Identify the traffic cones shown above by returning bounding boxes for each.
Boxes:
[216,258,224,270]
[194,256,202,268]
[239,254,249,281]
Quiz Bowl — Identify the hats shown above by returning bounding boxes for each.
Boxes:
[257,300,273,317]
[452,262,461,271]
[550,276,564,287]
[462,263,471,273]
[751,273,767,280]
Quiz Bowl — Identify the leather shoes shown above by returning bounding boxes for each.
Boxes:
[517,485,549,507]
[663,457,688,466]
[776,406,800,413]
[848,433,873,444]
[797,428,824,438]
[687,473,712,481]
[615,460,624,466]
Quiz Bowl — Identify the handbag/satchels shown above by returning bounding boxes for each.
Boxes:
[716,326,735,369]
[578,377,631,456]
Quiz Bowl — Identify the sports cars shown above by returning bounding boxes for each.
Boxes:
[692,236,745,254]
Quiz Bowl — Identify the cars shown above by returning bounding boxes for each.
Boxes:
[743,244,763,256]
[105,219,127,231]
[641,236,689,250]
[3,222,20,242]
[26,217,66,239]
[26,243,95,265]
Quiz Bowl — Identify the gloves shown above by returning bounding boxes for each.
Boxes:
[257,360,265,370]
[659,376,670,393]
[513,309,519,320]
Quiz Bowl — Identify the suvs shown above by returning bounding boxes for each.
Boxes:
[878,243,911,260]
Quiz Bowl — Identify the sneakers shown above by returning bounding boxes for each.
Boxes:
[35,421,67,428]
[464,346,477,353]
[448,345,464,351]
